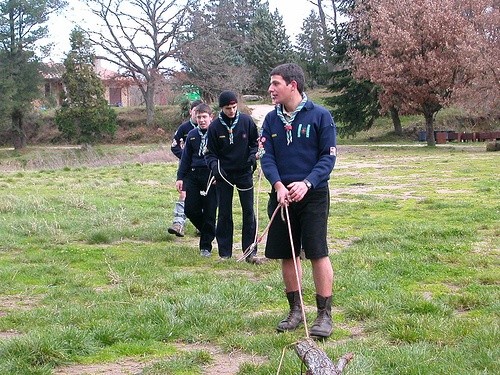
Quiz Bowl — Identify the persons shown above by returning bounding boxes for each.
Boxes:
[176,103,218,257]
[255,63,337,337]
[168,100,204,237]
[204,91,266,266]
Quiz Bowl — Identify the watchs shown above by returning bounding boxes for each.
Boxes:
[303,179,312,189]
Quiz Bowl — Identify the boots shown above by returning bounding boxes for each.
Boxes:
[308,294,335,337]
[276,290,306,332]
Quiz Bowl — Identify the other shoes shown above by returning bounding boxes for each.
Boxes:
[168,225,185,237]
[201,249,211,258]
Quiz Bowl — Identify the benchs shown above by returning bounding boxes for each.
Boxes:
[436,132,500,143]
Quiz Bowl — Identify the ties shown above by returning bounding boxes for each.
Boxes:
[197,126,208,156]
[277,91,308,146]
[190,117,197,129]
[218,111,240,144]
